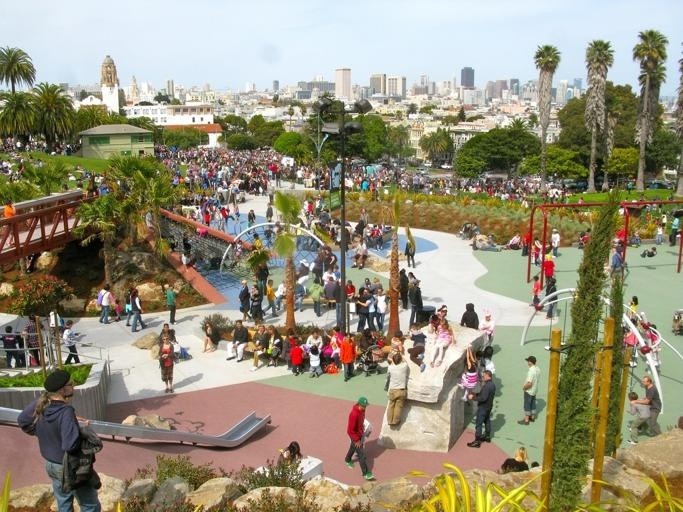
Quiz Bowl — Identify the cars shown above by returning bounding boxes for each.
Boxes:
[562,169,681,192]
[393,158,454,177]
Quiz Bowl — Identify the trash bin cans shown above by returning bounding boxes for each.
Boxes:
[421,306,436,323]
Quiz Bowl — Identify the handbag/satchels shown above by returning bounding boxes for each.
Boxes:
[125,303,133,314]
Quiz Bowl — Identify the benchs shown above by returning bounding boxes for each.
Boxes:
[243,329,287,368]
[280,296,336,311]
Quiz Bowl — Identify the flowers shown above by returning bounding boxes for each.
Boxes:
[1,271,75,321]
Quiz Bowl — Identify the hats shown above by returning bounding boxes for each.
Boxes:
[358,397,369,406]
[524,356,537,363]
[43,369,71,392]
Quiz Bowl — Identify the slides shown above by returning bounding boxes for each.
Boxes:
[0,408,271,448]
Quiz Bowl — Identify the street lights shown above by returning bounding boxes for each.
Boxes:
[314,92,372,337]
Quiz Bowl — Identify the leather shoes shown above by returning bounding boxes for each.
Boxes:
[468,442,480,447]
[481,436,491,442]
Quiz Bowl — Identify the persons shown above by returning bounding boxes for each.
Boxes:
[276,441,301,468]
[385,354,410,425]
[478,309,494,345]
[165,283,179,325]
[567,177,679,258]
[342,396,376,480]
[17,370,103,512]
[158,324,176,344]
[626,295,638,319]
[516,355,540,425]
[0,306,80,368]
[225,251,456,391]
[96,284,148,332]
[460,303,479,330]
[633,375,663,438]
[466,371,496,450]
[482,346,496,375]
[181,251,224,274]
[158,334,176,393]
[626,392,649,445]
[457,345,478,403]
[531,251,559,323]
[471,348,486,382]
[496,445,529,474]
[202,322,219,353]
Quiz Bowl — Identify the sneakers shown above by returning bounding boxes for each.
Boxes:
[225,356,356,382]
[362,473,374,480]
[346,460,353,470]
[417,354,424,360]
[517,420,528,425]
[435,360,441,367]
[628,427,642,433]
[420,364,426,372]
[626,439,637,445]
[529,417,535,422]
[430,361,434,369]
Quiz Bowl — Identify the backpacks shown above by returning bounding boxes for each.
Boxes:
[96,290,108,305]
